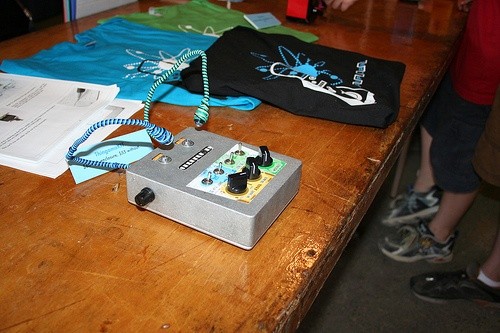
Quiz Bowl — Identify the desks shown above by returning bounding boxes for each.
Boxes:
[0,0,470,333]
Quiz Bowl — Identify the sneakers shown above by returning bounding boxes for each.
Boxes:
[380,179,443,226]
[378,221,459,264]
[407,264,500,308]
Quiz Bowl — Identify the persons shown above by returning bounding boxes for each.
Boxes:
[323,0,499,265]
[409,231,499,308]
